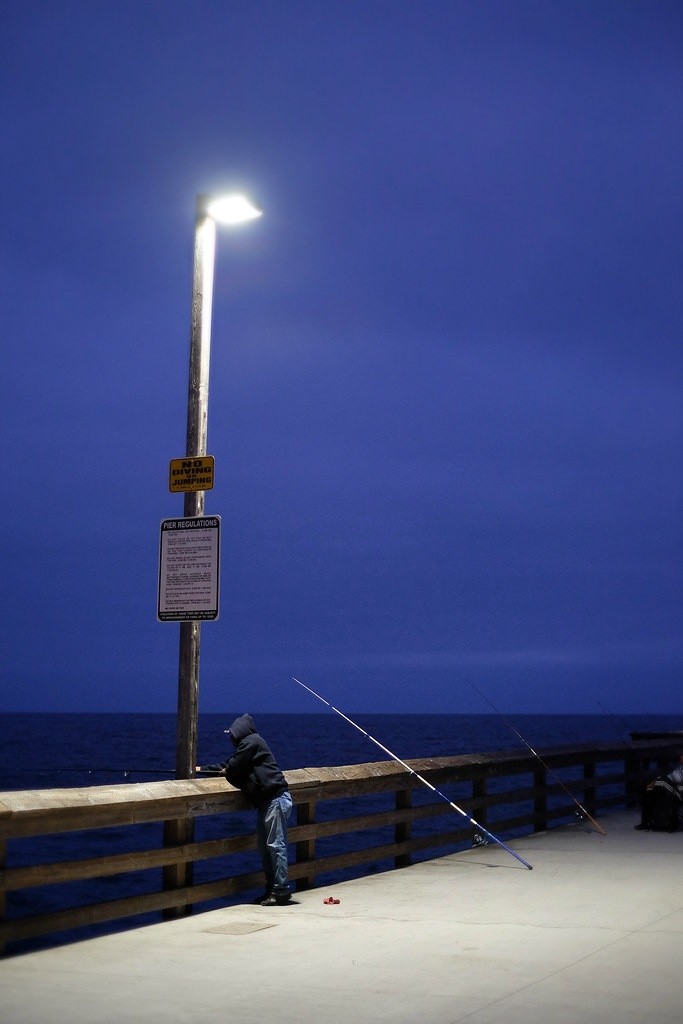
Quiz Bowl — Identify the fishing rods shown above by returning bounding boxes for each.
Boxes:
[292,675,534,872]
[471,683,607,834]
[68,767,226,776]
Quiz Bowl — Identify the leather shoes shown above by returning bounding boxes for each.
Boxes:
[634,824,648,830]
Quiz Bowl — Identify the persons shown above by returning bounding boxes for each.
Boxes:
[196,712,295,905]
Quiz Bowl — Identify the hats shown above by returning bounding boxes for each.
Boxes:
[223,729,231,734]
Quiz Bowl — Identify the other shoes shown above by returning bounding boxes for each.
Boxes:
[261,893,292,906]
[254,892,271,904]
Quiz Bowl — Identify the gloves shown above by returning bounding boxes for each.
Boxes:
[323,896,340,905]
